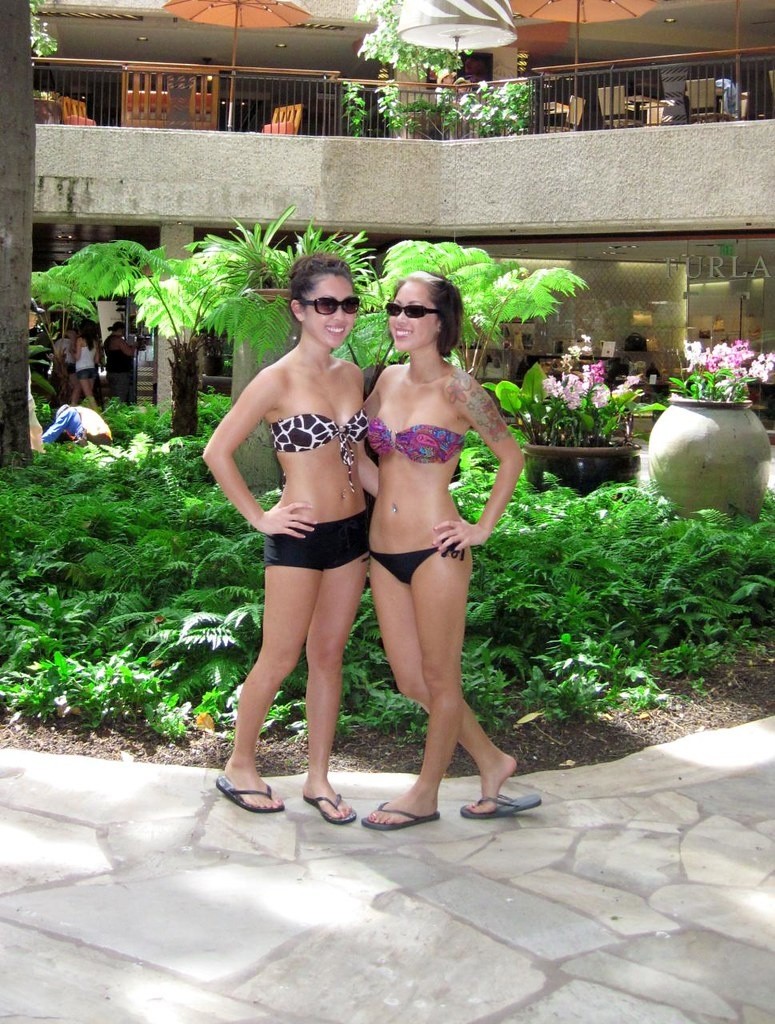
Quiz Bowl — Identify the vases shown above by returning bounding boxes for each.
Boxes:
[522,444,641,494]
[648,398,771,521]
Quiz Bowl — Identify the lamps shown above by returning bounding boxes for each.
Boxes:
[398,0,518,51]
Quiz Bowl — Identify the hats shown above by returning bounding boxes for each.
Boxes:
[437,67,457,84]
[453,77,469,85]
[29,299,47,316]
[107,321,126,331]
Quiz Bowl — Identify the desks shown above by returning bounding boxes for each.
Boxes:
[685,87,748,113]
[544,101,569,132]
[625,95,668,120]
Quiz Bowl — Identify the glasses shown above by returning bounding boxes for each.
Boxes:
[386,302,440,318]
[298,296,360,315]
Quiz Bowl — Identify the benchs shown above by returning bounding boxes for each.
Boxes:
[121,68,220,130]
[262,103,304,135]
[61,96,96,126]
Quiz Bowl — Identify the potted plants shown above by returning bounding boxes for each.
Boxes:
[32,90,63,124]
[393,98,451,139]
[188,203,384,496]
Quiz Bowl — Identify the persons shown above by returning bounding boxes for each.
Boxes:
[29,319,100,406]
[104,322,139,402]
[435,69,471,138]
[359,270,542,830]
[547,359,563,381]
[646,364,660,377]
[42,404,87,446]
[202,254,370,824]
[607,358,629,386]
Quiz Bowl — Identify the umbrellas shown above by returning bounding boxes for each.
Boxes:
[163,0,315,131]
[510,0,661,131]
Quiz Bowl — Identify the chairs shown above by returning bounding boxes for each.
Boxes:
[741,92,749,117]
[685,79,717,124]
[598,86,637,129]
[545,95,586,132]
[646,102,665,123]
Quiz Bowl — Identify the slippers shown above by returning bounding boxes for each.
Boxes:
[460,792,542,819]
[303,792,356,825]
[361,802,441,830]
[216,776,285,813]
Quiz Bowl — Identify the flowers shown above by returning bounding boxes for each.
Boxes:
[668,339,775,403]
[480,333,667,447]
